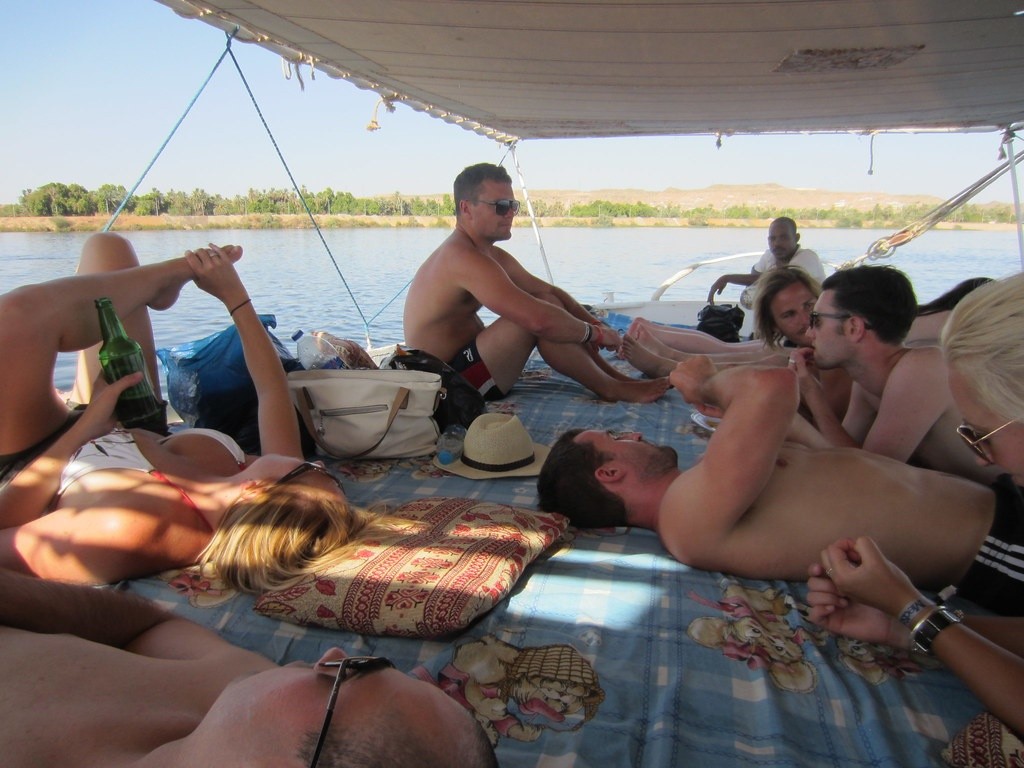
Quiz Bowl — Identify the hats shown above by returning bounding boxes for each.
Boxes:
[433,414,550,479]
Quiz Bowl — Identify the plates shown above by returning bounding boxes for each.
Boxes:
[690,410,720,431]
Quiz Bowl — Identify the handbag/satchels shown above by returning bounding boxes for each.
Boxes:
[287,370,446,460]
[386,344,484,428]
[156,314,315,458]
[698,303,744,342]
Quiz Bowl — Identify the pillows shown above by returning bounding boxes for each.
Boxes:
[256,501,571,637]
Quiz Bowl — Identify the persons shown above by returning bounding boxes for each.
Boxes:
[0,568,500,768]
[619,277,998,379]
[707,217,826,310]
[0,232,357,593]
[536,355,1024,618]
[404,163,670,404]
[752,264,852,421]
[788,265,1007,486]
[806,271,1024,738]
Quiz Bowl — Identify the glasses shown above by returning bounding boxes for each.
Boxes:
[276,462,346,496]
[957,416,1017,464]
[809,312,872,330]
[465,199,520,216]
[311,655,396,768]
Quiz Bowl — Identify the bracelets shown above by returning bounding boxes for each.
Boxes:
[230,299,251,316]
[898,596,964,654]
[580,322,593,343]
[593,325,603,345]
[591,318,601,325]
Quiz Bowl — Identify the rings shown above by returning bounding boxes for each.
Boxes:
[789,359,795,364]
[826,569,832,577]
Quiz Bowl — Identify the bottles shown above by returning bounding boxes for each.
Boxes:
[437,424,467,464]
[95,296,162,428]
[292,330,352,370]
